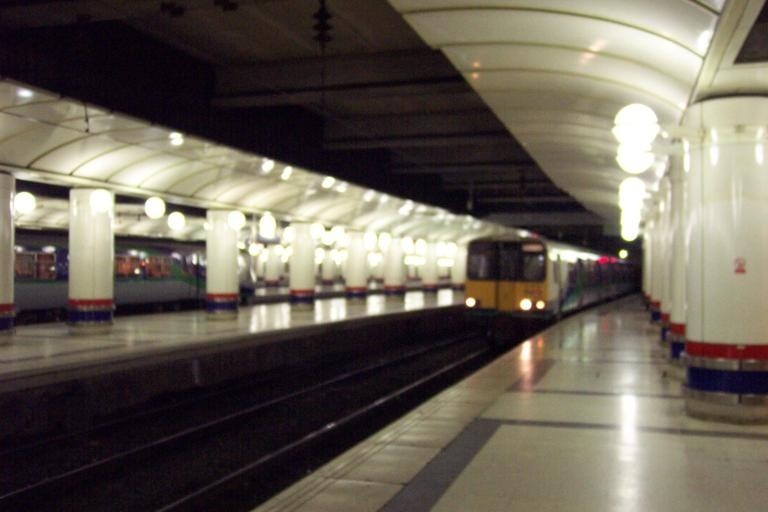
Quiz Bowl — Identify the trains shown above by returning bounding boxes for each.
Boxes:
[11,234,207,315]
[464,231,641,324]
